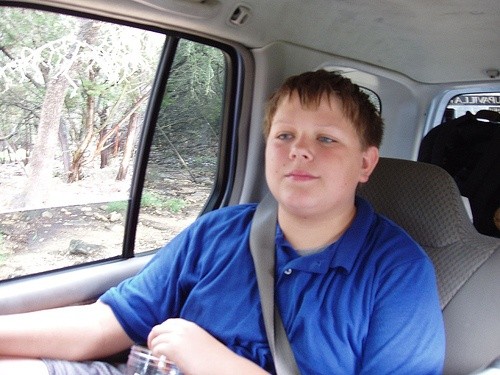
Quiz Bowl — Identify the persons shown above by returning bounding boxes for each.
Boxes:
[0,68,447,375]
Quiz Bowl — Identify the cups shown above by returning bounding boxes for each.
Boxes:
[125,343,182,374]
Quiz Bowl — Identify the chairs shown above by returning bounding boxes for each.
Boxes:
[355,156,500,375]
